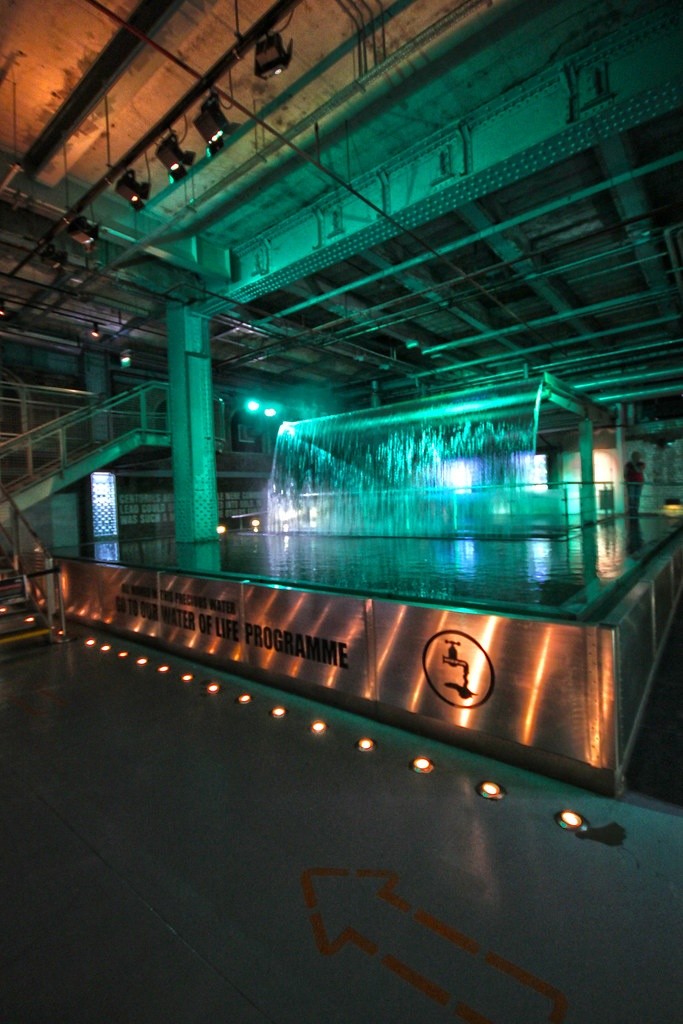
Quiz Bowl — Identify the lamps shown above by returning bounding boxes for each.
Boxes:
[66,217,98,254]
[192,86,242,156]
[154,126,196,184]
[37,244,68,274]
[253,29,293,80]
[92,322,99,338]
[114,167,150,212]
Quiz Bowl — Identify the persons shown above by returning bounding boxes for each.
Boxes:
[624,451,646,512]
